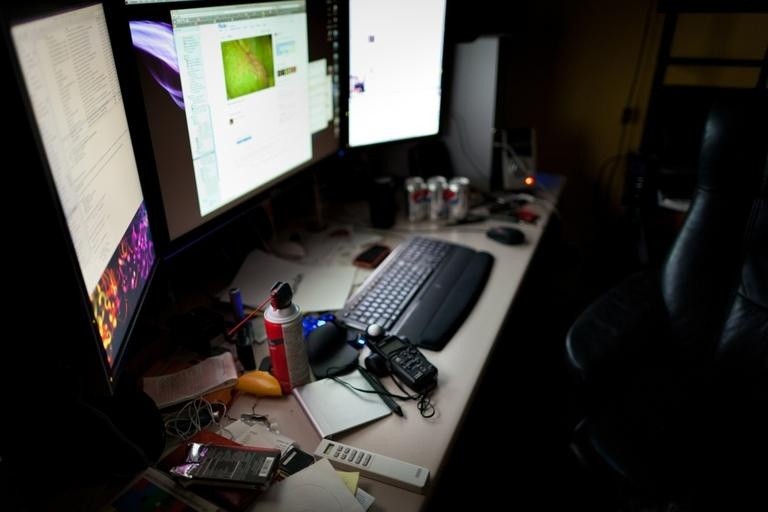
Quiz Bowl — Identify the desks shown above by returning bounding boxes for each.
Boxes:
[161,169,568,512]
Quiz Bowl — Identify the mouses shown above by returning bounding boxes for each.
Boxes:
[487,228,524,245]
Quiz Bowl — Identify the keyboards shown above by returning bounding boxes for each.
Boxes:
[336,236,494,351]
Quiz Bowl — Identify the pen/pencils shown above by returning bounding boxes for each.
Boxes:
[357,366,403,418]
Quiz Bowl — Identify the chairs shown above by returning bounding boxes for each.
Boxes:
[561,109,767,512]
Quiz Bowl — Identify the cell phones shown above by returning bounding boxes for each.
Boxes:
[352,244,390,269]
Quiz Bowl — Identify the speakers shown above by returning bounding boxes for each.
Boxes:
[408,142,454,182]
[107,391,166,473]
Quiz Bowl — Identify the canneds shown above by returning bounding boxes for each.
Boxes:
[442,176,471,221]
[403,176,428,223]
[425,175,449,223]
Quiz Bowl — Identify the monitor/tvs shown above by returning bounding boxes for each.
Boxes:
[114,0,347,281]
[0,0,163,397]
[343,0,454,154]
